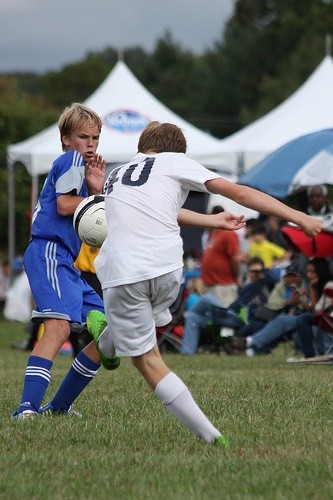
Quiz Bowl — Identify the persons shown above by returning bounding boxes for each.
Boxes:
[86,121,324,449]
[0,243,103,358]
[11,101,106,420]
[156,185,333,358]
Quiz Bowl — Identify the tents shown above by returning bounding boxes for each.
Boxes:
[205,56,333,214]
[7,61,235,268]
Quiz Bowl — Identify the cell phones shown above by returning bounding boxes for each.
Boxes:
[290,285,302,295]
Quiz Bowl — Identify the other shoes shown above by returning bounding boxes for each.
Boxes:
[224,336,255,356]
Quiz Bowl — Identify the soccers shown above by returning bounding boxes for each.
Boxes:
[72,195,108,247]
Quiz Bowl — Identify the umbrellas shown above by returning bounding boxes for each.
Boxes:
[237,129,333,199]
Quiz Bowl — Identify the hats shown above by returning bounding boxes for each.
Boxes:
[244,219,266,238]
[282,266,301,277]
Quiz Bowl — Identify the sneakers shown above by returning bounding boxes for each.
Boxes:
[86,310,121,370]
[11,404,39,419]
[38,403,72,417]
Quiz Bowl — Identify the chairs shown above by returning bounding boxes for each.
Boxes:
[311,288,333,356]
